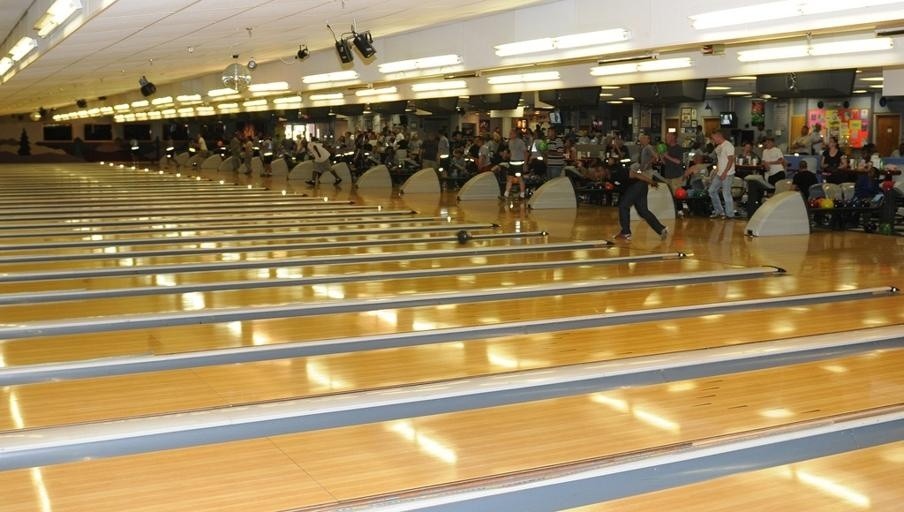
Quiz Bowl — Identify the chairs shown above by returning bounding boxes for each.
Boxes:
[564,145,904,234]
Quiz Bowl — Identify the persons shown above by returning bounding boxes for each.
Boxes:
[185,112,903,226]
[584,156,668,241]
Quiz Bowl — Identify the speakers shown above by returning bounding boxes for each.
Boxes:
[353,35,376,58]
[336,40,353,63]
[141,83,155,97]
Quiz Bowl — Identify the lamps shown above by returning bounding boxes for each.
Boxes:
[50,1,902,124]
[3,2,90,91]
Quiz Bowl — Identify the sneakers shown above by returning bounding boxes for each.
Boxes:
[659,225,673,247]
[709,213,732,222]
[497,193,525,202]
[612,230,632,244]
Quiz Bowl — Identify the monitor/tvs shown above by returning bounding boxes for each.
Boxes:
[720,112,738,128]
[548,111,562,126]
